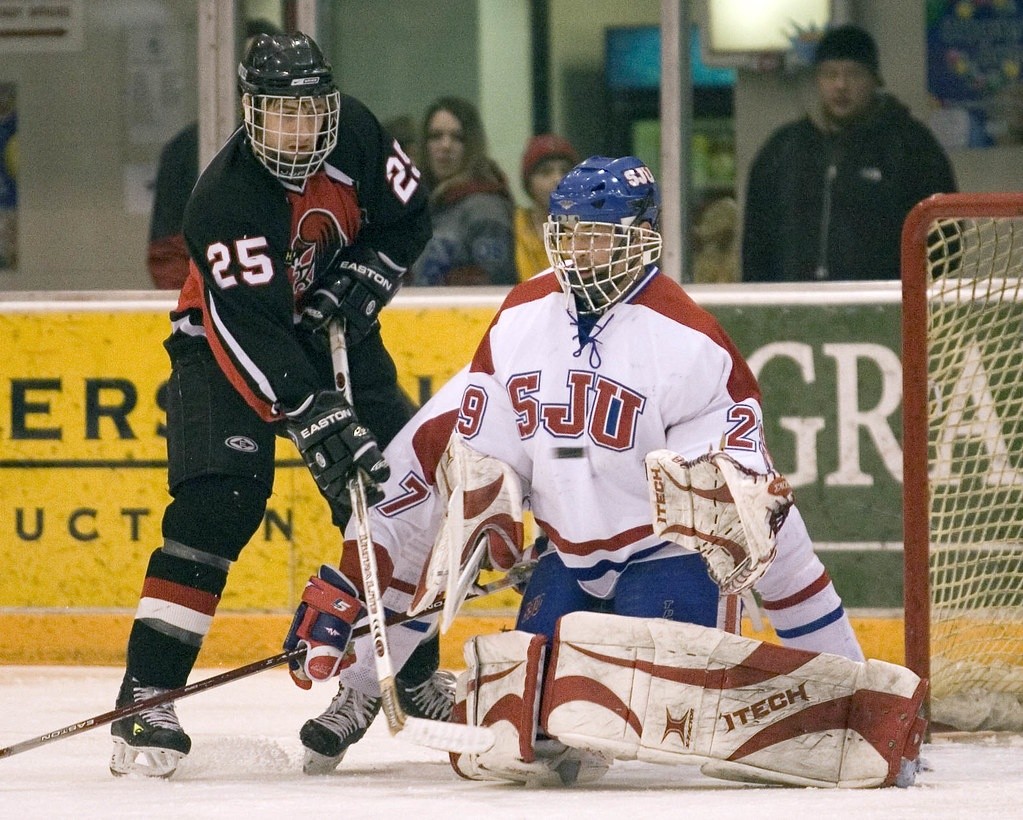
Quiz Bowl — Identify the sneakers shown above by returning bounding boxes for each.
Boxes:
[395,659,469,722]
[299,668,385,776]
[109,674,192,776]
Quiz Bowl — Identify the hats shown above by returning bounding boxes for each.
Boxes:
[812,24,886,88]
[523,133,583,192]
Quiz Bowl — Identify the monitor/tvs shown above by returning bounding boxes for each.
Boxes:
[606,22,737,120]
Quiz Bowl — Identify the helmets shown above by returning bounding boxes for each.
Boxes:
[547,157,660,301]
[237,31,337,181]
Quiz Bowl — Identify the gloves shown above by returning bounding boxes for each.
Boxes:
[281,564,369,690]
[285,388,390,526]
[298,243,403,358]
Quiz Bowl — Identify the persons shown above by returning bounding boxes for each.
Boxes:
[742,26,966,283]
[110,31,458,756]
[148,19,577,288]
[283,153,930,790]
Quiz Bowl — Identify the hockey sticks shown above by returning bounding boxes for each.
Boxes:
[0,565,536,759]
[327,316,494,755]
